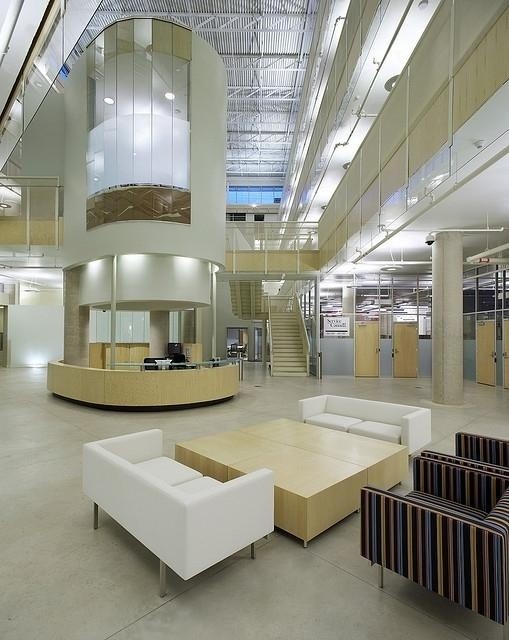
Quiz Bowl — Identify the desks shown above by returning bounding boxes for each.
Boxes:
[170,416,408,546]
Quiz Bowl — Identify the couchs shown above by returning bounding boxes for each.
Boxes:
[415,431,509,475]
[299,389,433,456]
[361,456,509,625]
[82,427,277,598]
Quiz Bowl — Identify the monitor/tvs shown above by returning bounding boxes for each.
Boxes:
[168,343,181,355]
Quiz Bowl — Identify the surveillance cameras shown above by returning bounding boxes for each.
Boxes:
[425,235,435,245]
